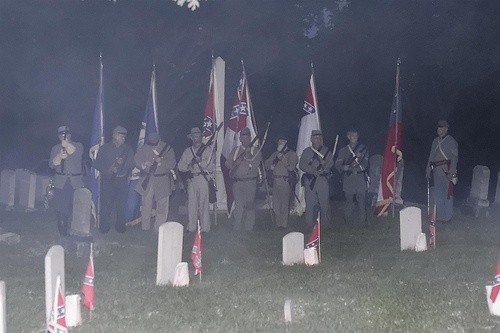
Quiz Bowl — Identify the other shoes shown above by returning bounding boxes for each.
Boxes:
[100,227,110,233]
[116,224,126,233]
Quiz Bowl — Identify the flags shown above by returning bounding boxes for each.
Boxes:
[304,218,320,249]
[46,277,68,333]
[124,72,159,227]
[190,226,203,275]
[489,253,500,303]
[83,61,103,219]
[292,72,325,216]
[80,247,96,311]
[373,61,404,215]
[199,61,263,219]
[427,206,437,243]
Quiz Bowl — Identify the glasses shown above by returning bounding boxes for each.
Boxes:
[57,129,71,135]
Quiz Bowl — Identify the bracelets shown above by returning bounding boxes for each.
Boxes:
[452,173,457,178]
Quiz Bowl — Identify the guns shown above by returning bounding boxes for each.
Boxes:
[183,121,224,179]
[142,126,182,190]
[112,128,138,179]
[56,114,70,176]
[231,114,274,173]
[338,136,377,180]
[309,124,344,190]
[265,124,299,180]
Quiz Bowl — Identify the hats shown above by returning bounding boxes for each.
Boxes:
[436,121,450,128]
[147,132,160,143]
[113,127,128,134]
[311,130,324,137]
[186,127,202,139]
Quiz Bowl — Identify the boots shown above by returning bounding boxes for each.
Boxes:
[57,214,68,237]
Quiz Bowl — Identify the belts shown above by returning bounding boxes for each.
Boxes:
[55,172,81,176]
[304,173,326,177]
[188,172,211,176]
[235,177,256,181]
[347,170,365,175]
[275,175,289,179]
[432,160,451,167]
[149,173,167,177]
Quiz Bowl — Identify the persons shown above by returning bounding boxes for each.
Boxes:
[336,127,369,228]
[299,129,334,240]
[133,129,177,239]
[94,126,136,233]
[48,125,84,237]
[265,135,298,232]
[423,118,460,222]
[175,127,211,236]
[224,128,262,235]
